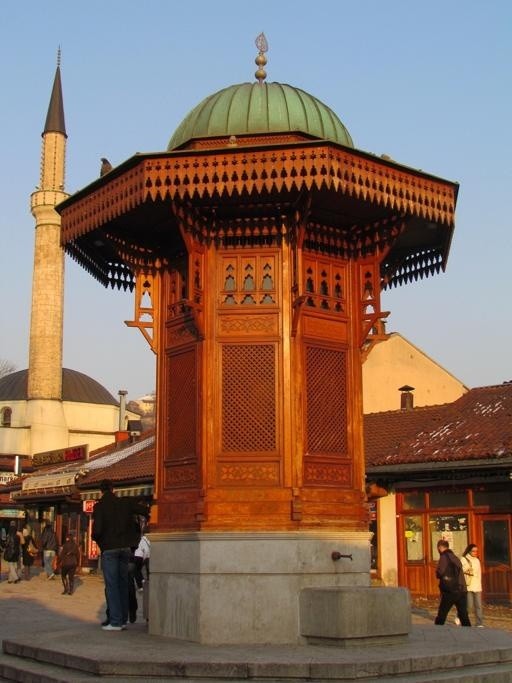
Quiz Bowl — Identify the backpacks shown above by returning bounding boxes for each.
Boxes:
[439,552,459,591]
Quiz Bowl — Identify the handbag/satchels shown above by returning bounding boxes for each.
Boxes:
[3,546,15,560]
[27,542,40,556]
[464,556,473,585]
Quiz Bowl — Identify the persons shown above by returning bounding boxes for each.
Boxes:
[91,479,152,629]
[434,539,472,626]
[454,544,487,631]
[4,519,82,596]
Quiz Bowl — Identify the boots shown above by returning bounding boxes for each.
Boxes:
[62,579,73,594]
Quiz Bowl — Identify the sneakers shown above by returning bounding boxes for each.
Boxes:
[101,610,137,631]
[455,611,460,625]
